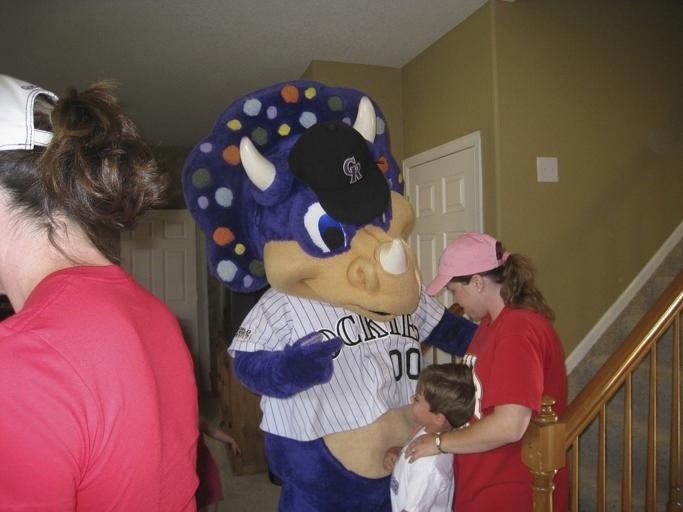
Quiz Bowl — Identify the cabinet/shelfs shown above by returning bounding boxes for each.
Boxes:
[213,335,268,476]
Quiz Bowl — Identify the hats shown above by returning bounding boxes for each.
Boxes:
[0,74,59,151]
[426,232,511,297]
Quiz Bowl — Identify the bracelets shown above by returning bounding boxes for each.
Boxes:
[433,431,448,454]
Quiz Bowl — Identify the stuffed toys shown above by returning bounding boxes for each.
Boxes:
[180,79,479,512]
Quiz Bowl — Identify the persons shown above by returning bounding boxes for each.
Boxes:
[197,415,243,512]
[404,231,572,512]
[0,72,200,512]
[382,363,476,512]
